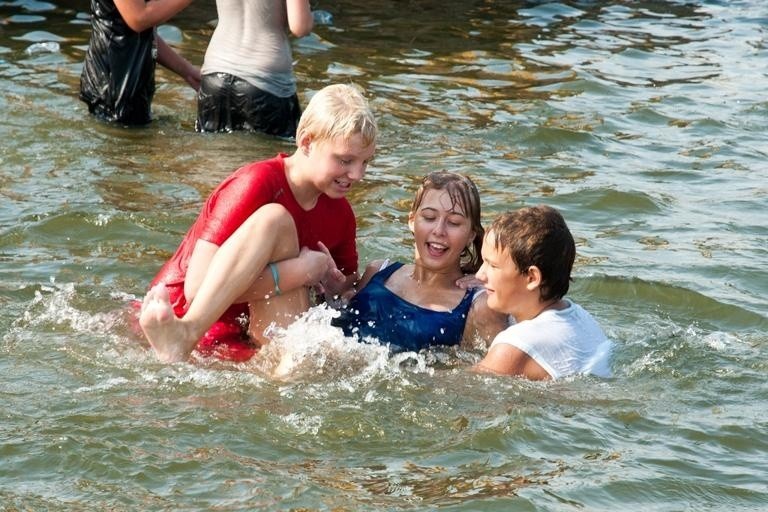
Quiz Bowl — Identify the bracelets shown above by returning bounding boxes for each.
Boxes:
[268,262,283,296]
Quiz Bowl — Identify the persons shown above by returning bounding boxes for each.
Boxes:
[78,0,202,129]
[131,84,377,364]
[454,204,616,383]
[194,0,316,140]
[138,169,511,364]
